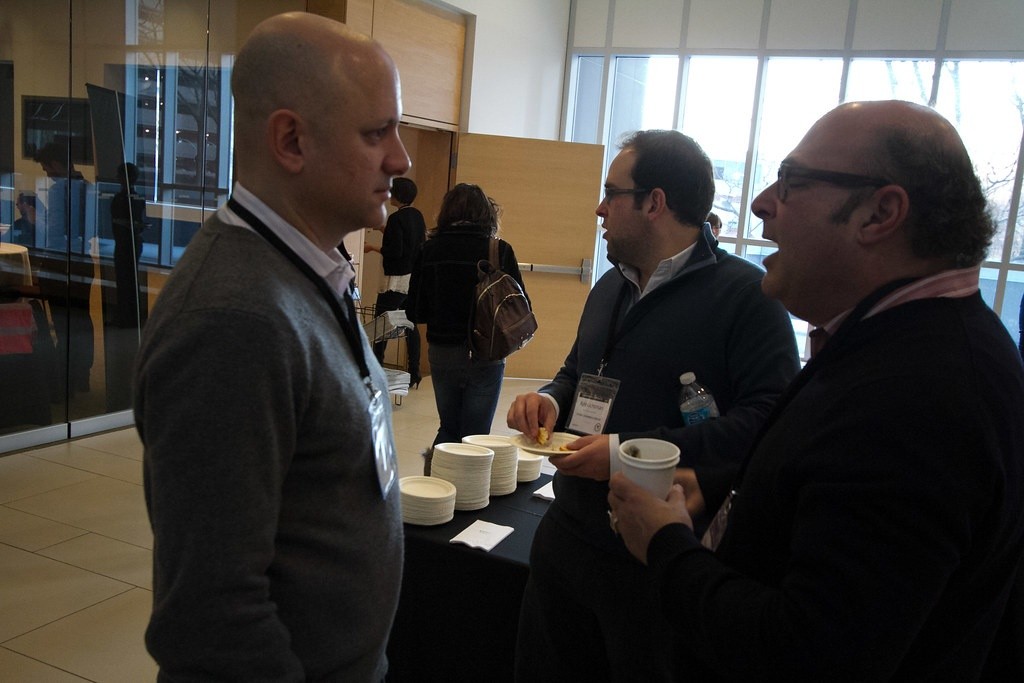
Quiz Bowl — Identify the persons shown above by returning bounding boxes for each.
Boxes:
[364,178,426,389]
[134,12,411,683]
[25,142,94,402]
[405,183,533,477]
[706,212,723,240]
[507,129,801,683]
[0,189,47,286]
[607,100,1024,683]
[110,163,147,329]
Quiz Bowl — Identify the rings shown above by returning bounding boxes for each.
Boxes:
[608,511,618,534]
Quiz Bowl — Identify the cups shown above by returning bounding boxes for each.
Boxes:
[619,437,681,502]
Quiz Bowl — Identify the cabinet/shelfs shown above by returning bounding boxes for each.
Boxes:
[353,303,408,406]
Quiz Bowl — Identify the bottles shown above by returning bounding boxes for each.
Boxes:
[678,372,720,428]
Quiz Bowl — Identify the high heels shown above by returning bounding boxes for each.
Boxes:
[407,371,422,390]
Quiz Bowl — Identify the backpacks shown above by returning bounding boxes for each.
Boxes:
[469,237,539,362]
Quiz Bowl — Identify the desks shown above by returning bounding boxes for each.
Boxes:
[386,473,558,683]
[0,242,35,304]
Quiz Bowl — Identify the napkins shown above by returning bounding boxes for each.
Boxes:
[532,480,555,501]
[449,519,515,552]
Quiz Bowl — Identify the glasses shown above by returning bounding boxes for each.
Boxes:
[776,164,888,202]
[604,186,671,209]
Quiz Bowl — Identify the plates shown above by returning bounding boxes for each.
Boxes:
[398,432,582,526]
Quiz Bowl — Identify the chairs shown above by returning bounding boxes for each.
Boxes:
[0,258,71,355]
